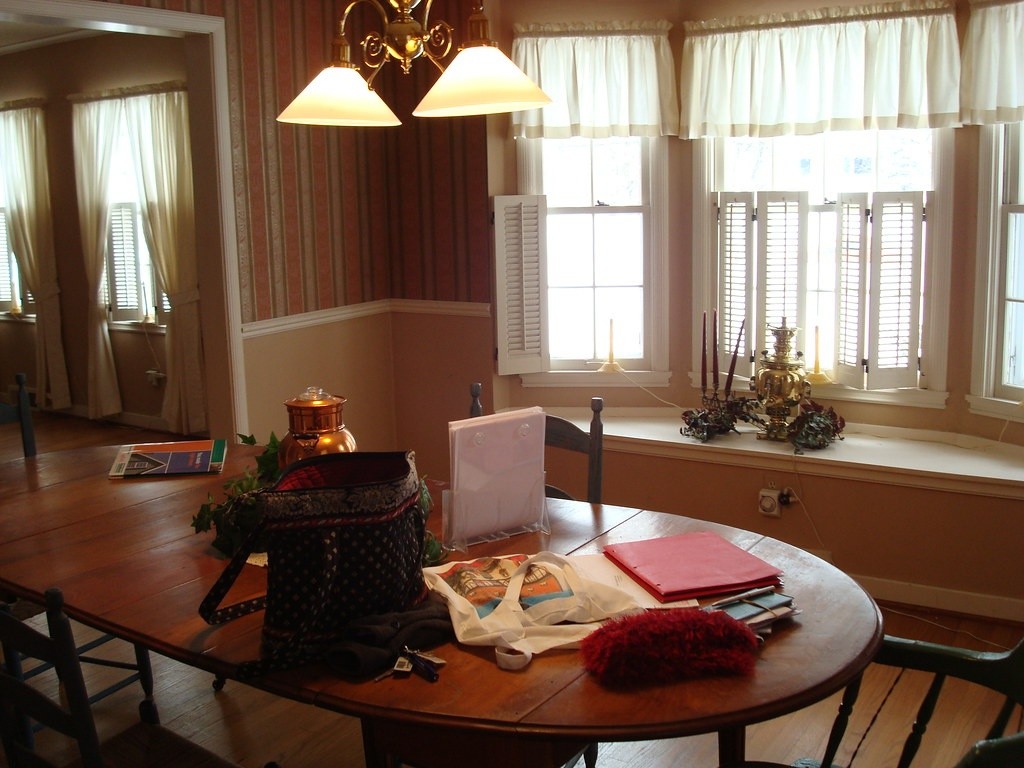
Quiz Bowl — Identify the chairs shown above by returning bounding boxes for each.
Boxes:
[0,582,239,767]
[740,632,1023,768]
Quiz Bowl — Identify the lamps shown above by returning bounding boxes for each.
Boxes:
[274,0,559,128]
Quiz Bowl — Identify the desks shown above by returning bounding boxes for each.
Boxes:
[0,436,883,767]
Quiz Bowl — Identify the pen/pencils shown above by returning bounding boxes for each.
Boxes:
[711,585,775,607]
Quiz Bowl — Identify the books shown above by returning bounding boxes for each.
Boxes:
[108,439,227,480]
[603,531,794,630]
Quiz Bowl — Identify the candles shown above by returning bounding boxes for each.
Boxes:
[725,317,747,390]
[812,326,821,372]
[712,307,719,381]
[607,317,613,363]
[702,311,708,385]
